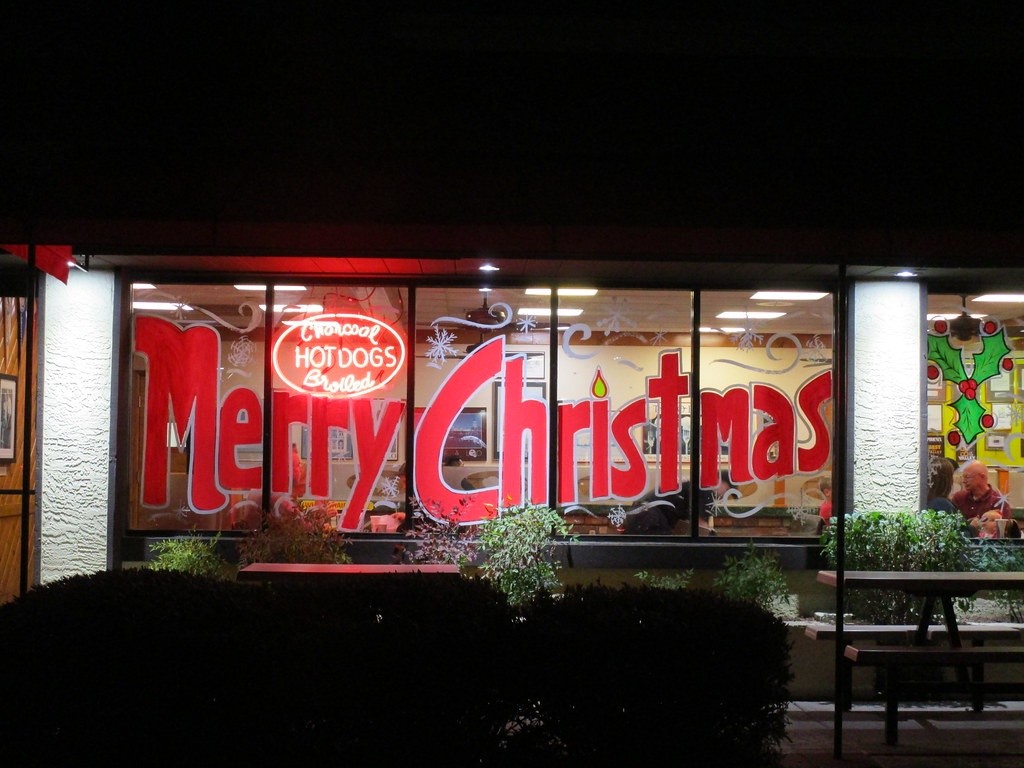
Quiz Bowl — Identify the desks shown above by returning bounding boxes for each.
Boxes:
[815,569,1024,693]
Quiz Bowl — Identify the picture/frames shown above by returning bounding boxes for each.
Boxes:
[494,381,547,459]
[927,403,943,433]
[0,373,18,464]
[985,366,1014,404]
[300,424,354,461]
[927,362,948,402]
[372,398,398,461]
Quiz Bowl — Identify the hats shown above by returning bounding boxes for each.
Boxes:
[718,469,738,487]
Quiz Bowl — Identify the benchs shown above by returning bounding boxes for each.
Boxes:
[842,644,1024,746]
[804,622,1024,712]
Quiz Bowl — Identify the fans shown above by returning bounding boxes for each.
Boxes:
[415,290,571,334]
[935,293,1024,341]
[416,334,544,360]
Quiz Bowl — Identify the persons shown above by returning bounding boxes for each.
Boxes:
[817,477,833,535]
[926,457,977,538]
[444,455,476,533]
[951,462,1013,539]
[620,469,738,535]
[382,463,443,533]
